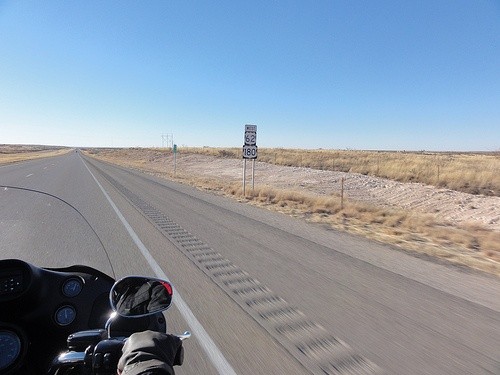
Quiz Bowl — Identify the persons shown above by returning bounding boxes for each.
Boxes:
[116,330,182,375]
[112,278,172,316]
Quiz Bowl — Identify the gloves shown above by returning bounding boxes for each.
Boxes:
[117,329,182,375]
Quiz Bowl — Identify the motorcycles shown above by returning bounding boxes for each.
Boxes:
[0,185,192,375]
